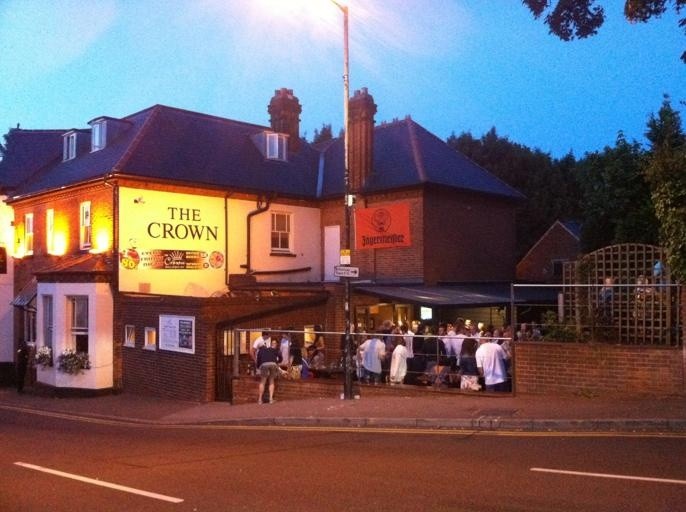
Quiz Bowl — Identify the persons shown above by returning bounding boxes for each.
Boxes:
[598,272,676,342]
[250,324,325,403]
[341,317,541,392]
[15,340,29,394]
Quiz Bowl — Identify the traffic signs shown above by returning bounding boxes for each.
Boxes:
[334,266,362,278]
